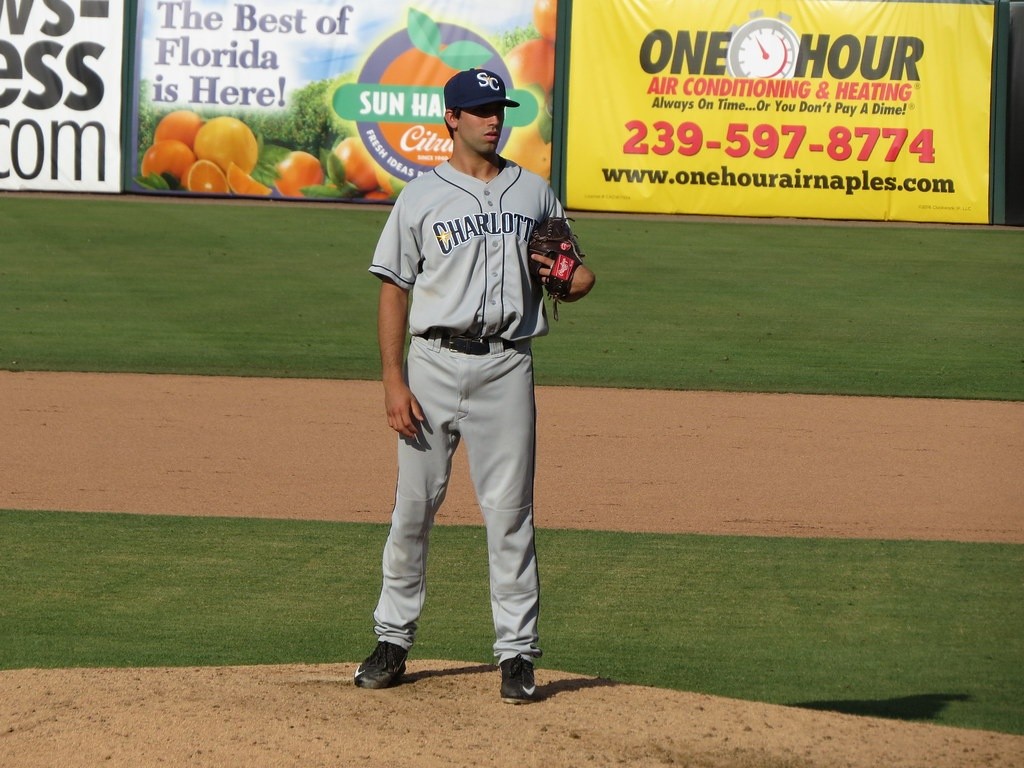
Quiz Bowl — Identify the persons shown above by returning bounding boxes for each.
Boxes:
[354,67,595,704]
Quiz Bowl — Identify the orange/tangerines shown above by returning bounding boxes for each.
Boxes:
[140,111,389,199]
[496,0,558,182]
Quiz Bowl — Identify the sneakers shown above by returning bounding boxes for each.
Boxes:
[501,654,536,704]
[354,642,408,689]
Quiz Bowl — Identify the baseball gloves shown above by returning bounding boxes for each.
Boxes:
[527,216,586,303]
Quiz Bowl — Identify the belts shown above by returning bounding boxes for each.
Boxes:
[417,327,515,355]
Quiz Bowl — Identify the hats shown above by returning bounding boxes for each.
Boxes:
[444,68,520,110]
